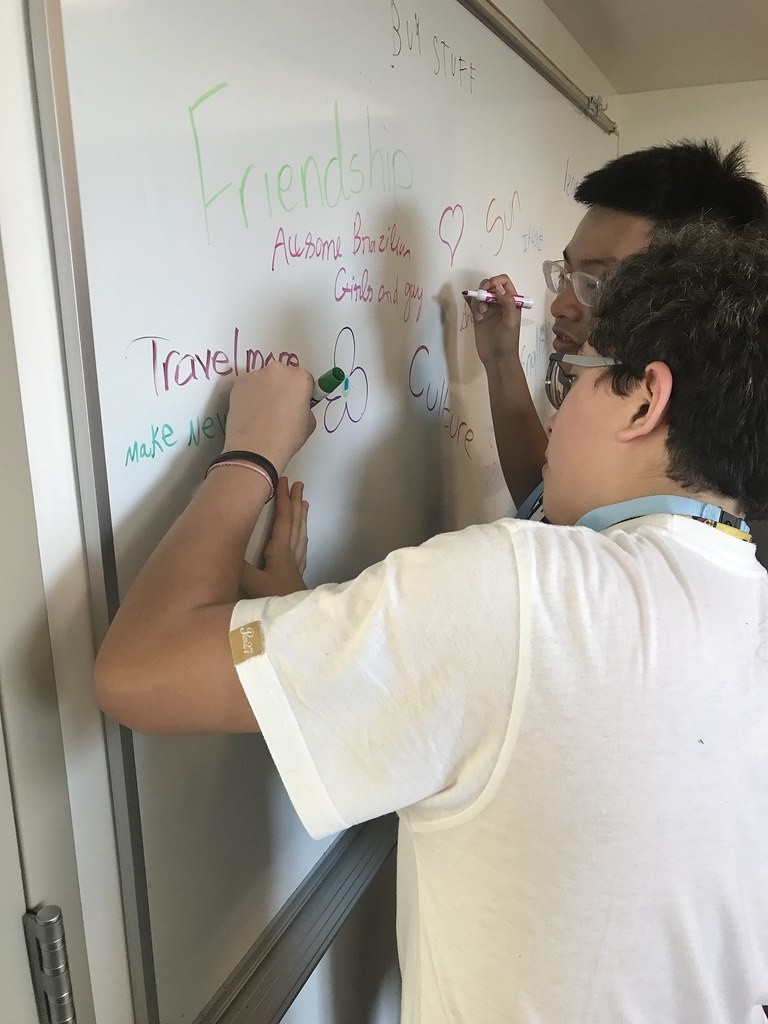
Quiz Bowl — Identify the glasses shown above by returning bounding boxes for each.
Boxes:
[542,259,608,308]
[546,353,651,410]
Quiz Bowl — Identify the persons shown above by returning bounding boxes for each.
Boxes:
[89,208,768,1024]
[461,134,768,567]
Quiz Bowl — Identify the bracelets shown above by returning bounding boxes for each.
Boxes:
[202,449,280,503]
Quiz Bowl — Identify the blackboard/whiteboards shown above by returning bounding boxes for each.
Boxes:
[0,0,621,1024]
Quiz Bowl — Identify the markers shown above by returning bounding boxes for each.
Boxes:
[462,289,534,311]
[310,367,345,409]
